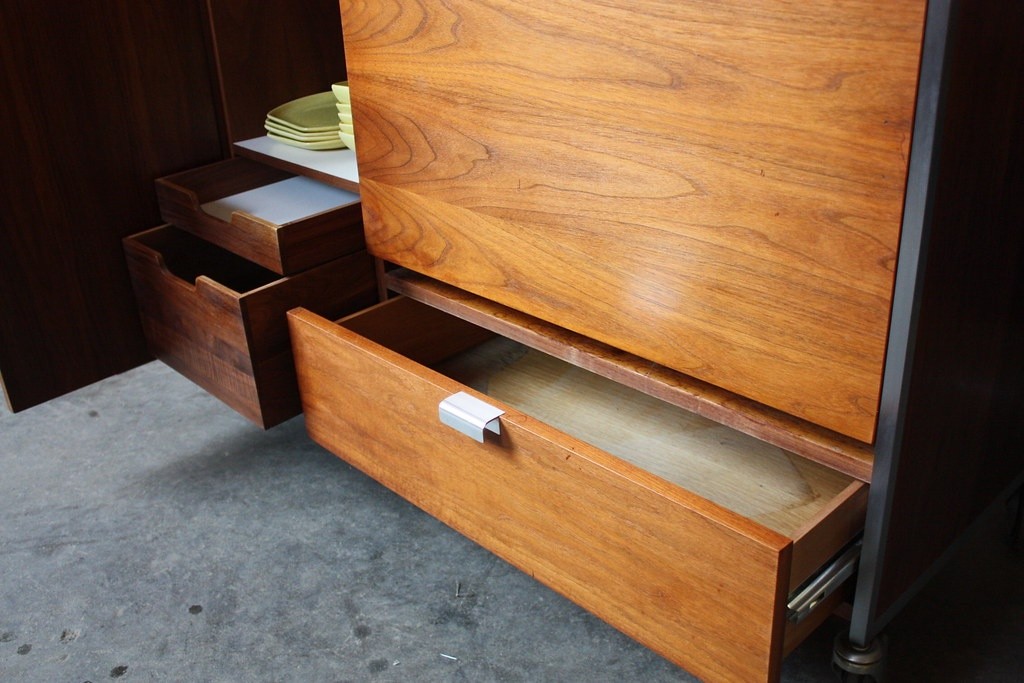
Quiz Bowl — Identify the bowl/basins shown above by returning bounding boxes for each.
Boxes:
[331,80,356,152]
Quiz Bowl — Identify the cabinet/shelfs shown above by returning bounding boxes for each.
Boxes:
[119,1,1024,682]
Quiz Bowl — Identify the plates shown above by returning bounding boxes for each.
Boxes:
[264,80,345,154]
